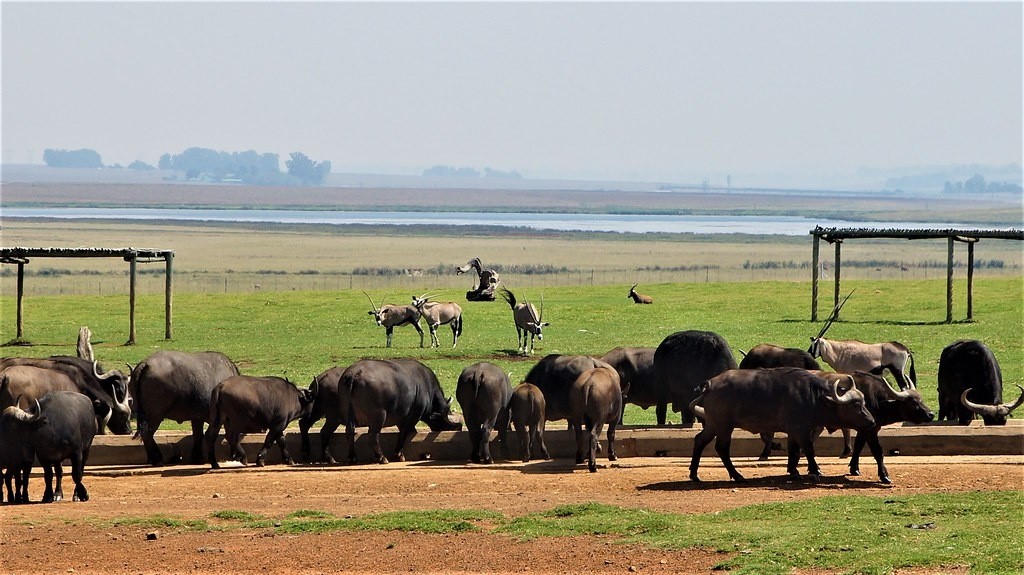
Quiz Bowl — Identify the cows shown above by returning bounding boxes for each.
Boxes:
[0,329,1024,506]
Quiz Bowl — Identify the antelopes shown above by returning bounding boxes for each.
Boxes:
[806,290,917,392]
[626,284,656,304]
[363,290,550,355]
